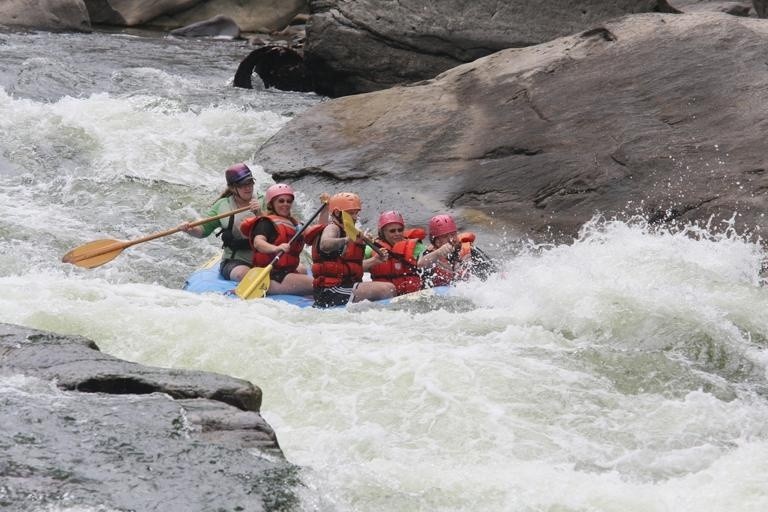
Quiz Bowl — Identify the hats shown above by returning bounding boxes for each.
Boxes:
[236,175,256,186]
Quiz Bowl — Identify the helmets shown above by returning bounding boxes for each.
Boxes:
[429,214,457,238]
[266,183,294,210]
[379,211,404,228]
[225,163,251,184]
[329,192,362,216]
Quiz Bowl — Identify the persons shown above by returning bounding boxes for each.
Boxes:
[362,211,426,296]
[416,214,500,286]
[303,192,398,307]
[178,163,267,281]
[240,183,330,294]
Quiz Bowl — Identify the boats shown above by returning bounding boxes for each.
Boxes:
[184,251,458,313]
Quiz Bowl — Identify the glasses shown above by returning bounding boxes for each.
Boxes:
[272,197,292,204]
[384,228,403,233]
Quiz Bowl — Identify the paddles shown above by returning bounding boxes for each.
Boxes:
[342,211,387,262]
[62,199,258,268]
[235,195,331,300]
